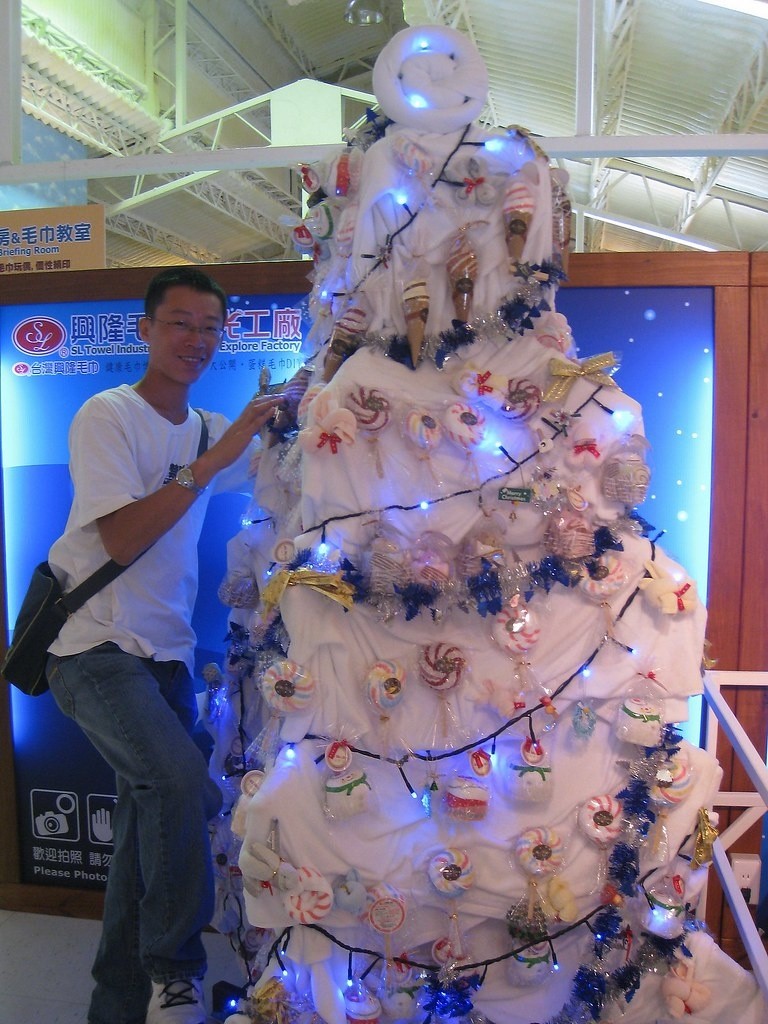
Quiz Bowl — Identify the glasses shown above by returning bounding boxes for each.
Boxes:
[146,316,226,339]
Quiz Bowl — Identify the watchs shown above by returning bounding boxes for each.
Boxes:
[175,468,206,495]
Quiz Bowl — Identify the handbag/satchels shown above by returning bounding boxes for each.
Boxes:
[1,559,73,697]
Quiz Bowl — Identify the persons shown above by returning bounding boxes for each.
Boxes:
[43,265,288,1024]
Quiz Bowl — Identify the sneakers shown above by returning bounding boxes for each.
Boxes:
[146,973,208,1024]
